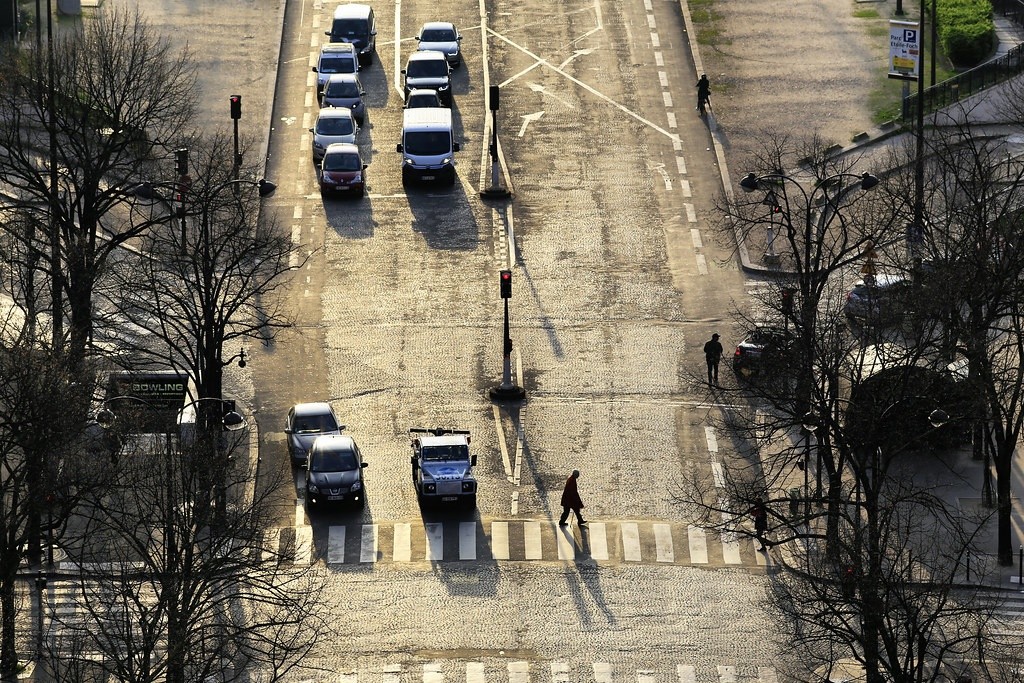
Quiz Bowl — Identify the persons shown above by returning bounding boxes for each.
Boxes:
[696,74,712,111]
[751,498,773,551]
[704,333,723,383]
[559,470,587,526]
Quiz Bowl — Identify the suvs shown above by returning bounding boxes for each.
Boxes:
[408,427,478,507]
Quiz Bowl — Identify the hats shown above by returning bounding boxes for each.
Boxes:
[712,334,721,337]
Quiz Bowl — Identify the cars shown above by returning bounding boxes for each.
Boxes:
[843,274,929,326]
[284,403,347,471]
[402,88,446,108]
[301,434,369,514]
[309,107,361,169]
[316,142,368,200]
[733,331,787,373]
[415,21,463,69]
[319,73,366,127]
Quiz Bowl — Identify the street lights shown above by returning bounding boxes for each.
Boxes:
[131,179,279,513]
[802,395,951,626]
[737,169,883,462]
[93,395,248,683]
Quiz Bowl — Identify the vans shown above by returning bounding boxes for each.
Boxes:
[312,42,363,104]
[400,49,455,104]
[395,106,460,189]
[325,3,377,66]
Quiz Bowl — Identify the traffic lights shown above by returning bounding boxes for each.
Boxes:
[229,94,242,119]
[499,269,512,299]
[173,147,189,175]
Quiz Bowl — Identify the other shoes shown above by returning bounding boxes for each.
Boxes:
[709,380,712,384]
[559,522,568,525]
[714,378,718,381]
[696,107,699,111]
[578,521,587,525]
